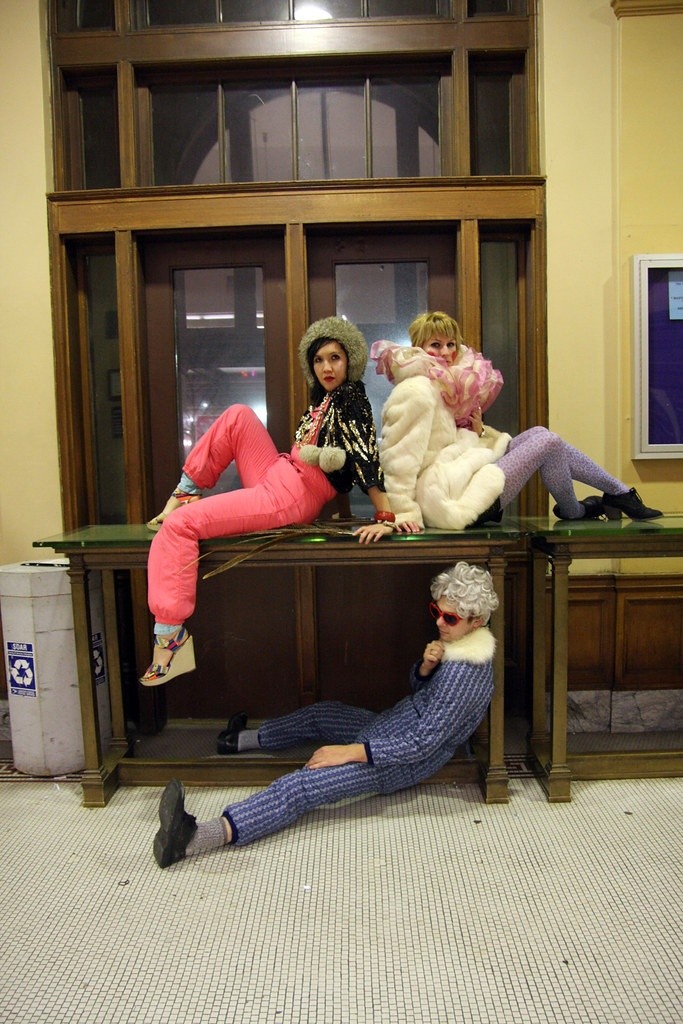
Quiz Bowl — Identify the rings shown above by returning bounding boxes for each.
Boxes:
[430,649,434,654]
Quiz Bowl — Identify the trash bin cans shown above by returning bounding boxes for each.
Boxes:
[0,557,89,778]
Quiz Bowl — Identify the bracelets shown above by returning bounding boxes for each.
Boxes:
[375,511,397,530]
[480,427,485,437]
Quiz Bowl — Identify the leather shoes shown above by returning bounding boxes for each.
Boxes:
[217,711,251,755]
[153,777,198,869]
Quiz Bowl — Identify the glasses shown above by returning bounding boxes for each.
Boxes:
[429,600,463,626]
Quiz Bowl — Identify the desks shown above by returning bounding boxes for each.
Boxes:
[31,524,532,809]
[503,516,682,802]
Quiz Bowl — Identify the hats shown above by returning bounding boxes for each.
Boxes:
[300,317,370,407]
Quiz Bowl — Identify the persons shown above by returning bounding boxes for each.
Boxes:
[140,317,397,686]
[371,312,665,534]
[153,560,498,868]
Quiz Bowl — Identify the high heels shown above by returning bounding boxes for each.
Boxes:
[553,496,606,520]
[146,488,203,531]
[602,486,663,521]
[139,628,196,686]
